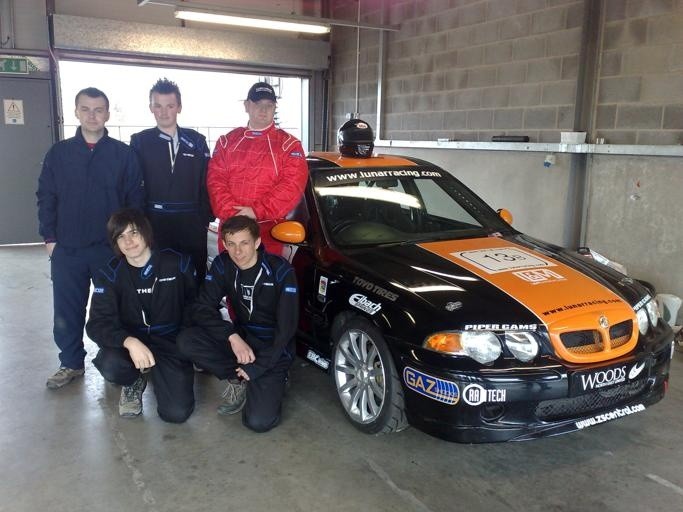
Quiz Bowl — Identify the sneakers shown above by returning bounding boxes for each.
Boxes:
[117,375,148,418]
[215,377,249,416]
[45,361,86,391]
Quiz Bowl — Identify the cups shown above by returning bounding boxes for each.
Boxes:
[595,138,603,145]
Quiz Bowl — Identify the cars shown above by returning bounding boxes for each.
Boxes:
[204,152,675,444]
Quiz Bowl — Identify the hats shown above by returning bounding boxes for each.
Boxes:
[246,81,278,103]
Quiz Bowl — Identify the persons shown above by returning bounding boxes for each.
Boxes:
[130,76,215,277]
[35,88,147,389]
[176,216,301,433]
[207,82,309,323]
[85,208,200,424]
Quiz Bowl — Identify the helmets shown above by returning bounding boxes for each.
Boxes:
[336,118,375,159]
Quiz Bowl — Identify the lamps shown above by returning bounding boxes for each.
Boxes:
[169,7,332,37]
